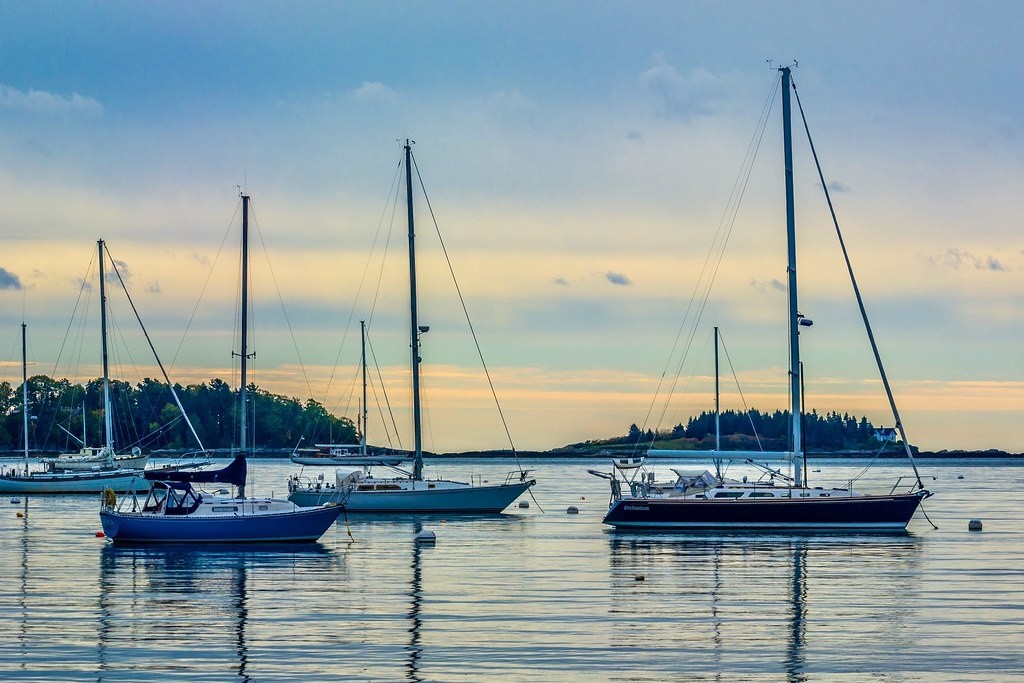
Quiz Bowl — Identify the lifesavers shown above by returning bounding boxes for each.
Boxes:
[631,482,647,498]
[103,488,116,507]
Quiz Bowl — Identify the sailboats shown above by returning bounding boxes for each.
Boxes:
[0,237,215,498]
[586,61,936,529]
[283,140,537,515]
[285,321,413,466]
[97,184,360,545]
